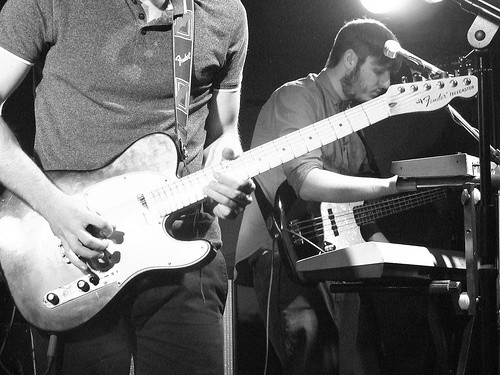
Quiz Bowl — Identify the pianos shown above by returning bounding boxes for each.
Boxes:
[389,153,500,183]
[296,240,499,286]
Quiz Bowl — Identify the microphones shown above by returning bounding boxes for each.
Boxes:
[383,39,448,77]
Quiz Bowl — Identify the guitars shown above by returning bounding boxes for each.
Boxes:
[274,176,470,286]
[0,67,480,333]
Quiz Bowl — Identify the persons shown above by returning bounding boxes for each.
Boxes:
[234,18,402,375]
[0,0,255,375]
